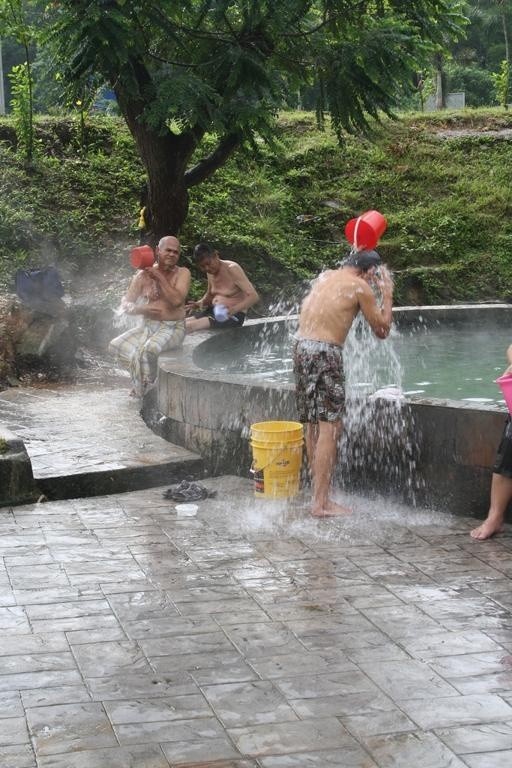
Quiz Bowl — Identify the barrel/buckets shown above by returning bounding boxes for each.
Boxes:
[250,420,303,500]
[493,373,512,414]
[345,210,388,251]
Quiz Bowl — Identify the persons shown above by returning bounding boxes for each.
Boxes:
[105,234,193,398]
[184,241,259,335]
[292,243,396,517]
[468,343,512,541]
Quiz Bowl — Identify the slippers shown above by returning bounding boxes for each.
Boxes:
[162,479,216,501]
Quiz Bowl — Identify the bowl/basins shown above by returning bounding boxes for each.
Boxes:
[175,503,198,517]
